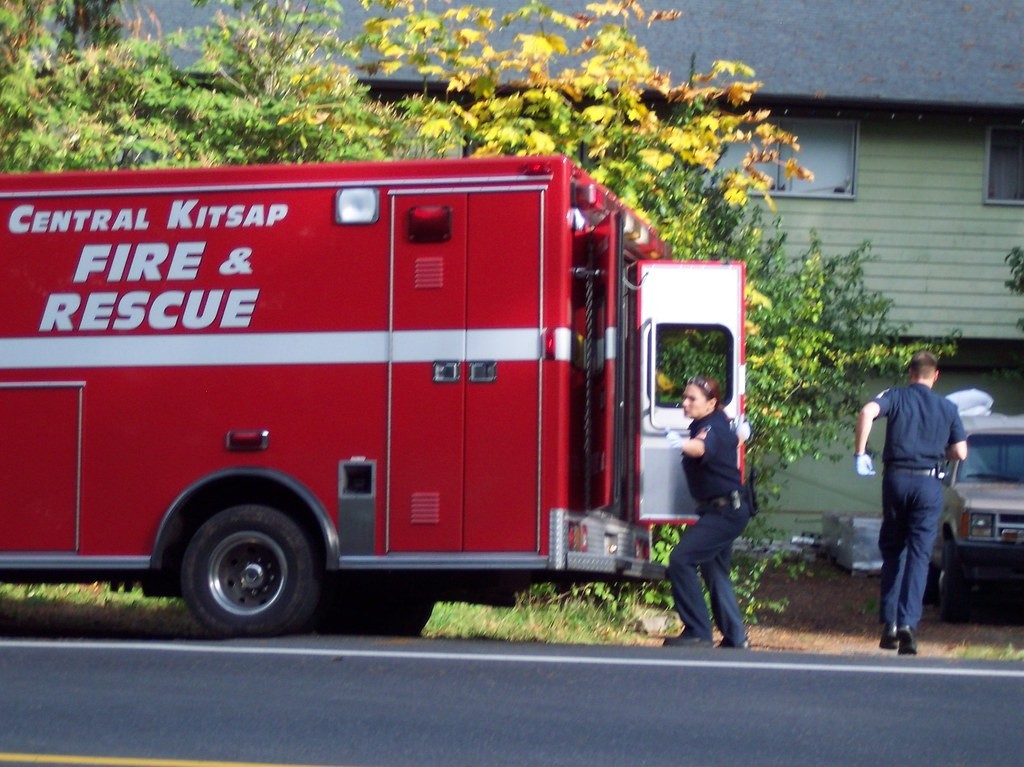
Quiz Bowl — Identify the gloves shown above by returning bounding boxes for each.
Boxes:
[666,431,688,453]
[737,421,752,440]
[853,451,876,476]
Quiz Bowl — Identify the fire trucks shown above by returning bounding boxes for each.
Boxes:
[2,152,748,639]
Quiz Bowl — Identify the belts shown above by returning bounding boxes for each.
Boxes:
[697,497,731,506]
[896,467,945,480]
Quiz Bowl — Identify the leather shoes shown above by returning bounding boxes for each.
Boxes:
[896,625,917,654]
[879,623,897,650]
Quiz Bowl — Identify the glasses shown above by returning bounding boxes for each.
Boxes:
[687,377,715,398]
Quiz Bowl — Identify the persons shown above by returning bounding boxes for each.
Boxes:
[853,351,969,656]
[662,376,751,649]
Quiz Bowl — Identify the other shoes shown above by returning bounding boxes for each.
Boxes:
[718,636,749,649]
[664,634,714,648]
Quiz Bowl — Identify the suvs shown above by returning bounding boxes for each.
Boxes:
[927,413,1024,624]
